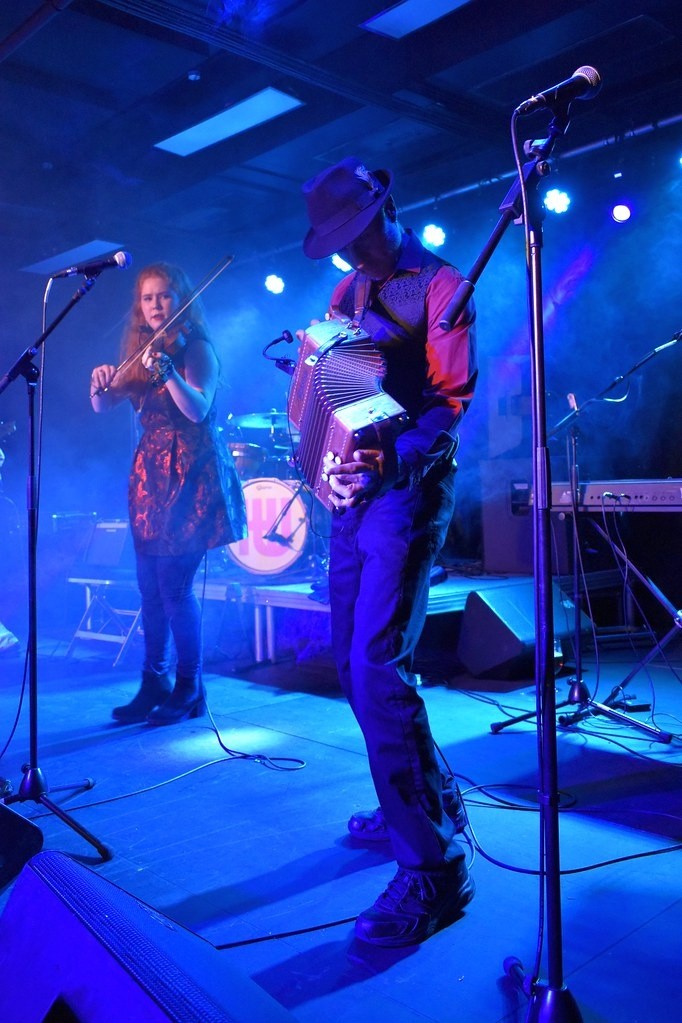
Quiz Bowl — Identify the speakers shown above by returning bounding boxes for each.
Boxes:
[456,583,598,680]
[1,803,43,892]
[83,519,136,569]
[0,849,299,1023]
[481,456,611,576]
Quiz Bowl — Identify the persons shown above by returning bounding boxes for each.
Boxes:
[289,155,476,946]
[90,263,248,726]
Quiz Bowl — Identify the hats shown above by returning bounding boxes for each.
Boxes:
[300,157,394,259]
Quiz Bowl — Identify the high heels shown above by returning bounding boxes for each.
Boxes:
[112,671,172,722]
[145,666,206,723]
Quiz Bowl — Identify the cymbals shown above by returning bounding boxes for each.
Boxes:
[274,441,299,449]
[226,412,292,429]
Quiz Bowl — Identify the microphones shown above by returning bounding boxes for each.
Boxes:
[515,65,603,116]
[51,251,133,279]
[273,330,294,345]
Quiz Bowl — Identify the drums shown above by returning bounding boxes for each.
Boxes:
[226,440,268,481]
[225,476,326,577]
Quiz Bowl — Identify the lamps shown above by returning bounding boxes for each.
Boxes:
[542,176,590,214]
[423,213,458,250]
[329,248,355,282]
[261,270,286,299]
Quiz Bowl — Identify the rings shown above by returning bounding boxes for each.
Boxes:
[97,368,102,373]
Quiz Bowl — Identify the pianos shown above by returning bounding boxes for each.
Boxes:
[509,476,682,726]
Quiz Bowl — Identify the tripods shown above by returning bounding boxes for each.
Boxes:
[266,481,328,582]
[0,262,115,861]
[490,333,682,743]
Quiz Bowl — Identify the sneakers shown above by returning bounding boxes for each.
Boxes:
[353,859,476,947]
[348,785,470,840]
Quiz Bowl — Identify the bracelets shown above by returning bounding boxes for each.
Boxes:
[87,376,98,390]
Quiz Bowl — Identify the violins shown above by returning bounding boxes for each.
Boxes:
[146,318,193,389]
